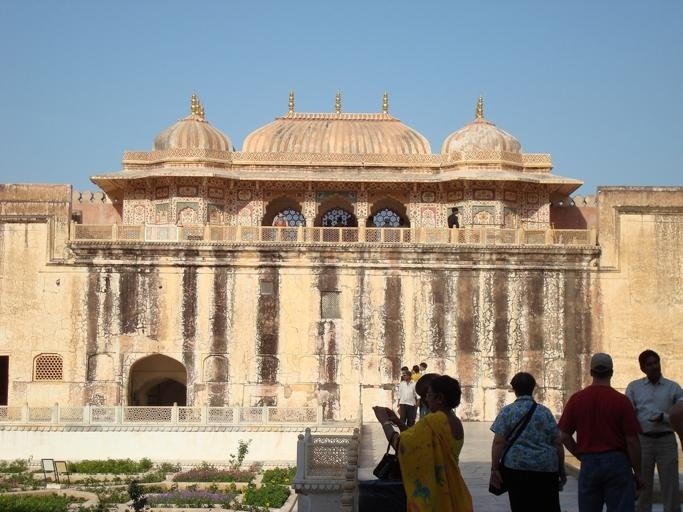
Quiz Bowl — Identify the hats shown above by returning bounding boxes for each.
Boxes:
[590,353,613,374]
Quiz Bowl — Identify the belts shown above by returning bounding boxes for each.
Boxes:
[641,431,674,439]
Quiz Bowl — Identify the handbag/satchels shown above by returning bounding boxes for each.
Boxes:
[489,463,508,496]
[373,453,402,481]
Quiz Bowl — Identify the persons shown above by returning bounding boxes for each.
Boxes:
[489,372,567,512]
[625,350,683,511]
[557,353,646,511]
[670,396,682,450]
[372,363,475,512]
[448,208,459,228]
[272,211,390,227]
